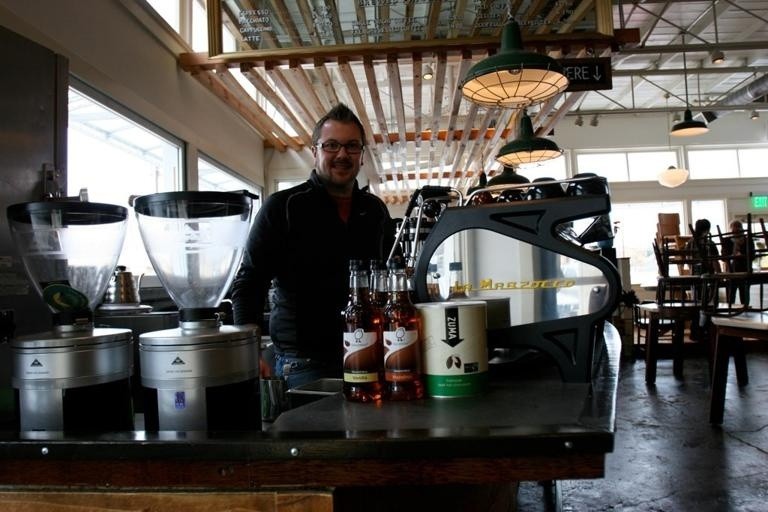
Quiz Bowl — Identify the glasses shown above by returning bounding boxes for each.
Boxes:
[316,139,365,154]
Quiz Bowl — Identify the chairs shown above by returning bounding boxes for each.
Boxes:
[629,214,768,429]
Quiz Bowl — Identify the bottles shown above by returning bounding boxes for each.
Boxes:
[380,256,426,402]
[342,258,387,403]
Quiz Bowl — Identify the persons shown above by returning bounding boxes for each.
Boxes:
[722,221,755,305]
[231,104,406,392]
[686,219,721,340]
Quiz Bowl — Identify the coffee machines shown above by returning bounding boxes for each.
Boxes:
[130,185,263,440]
[5,188,138,439]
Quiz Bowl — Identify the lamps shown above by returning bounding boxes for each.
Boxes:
[670,1,728,136]
[657,112,689,189]
[748,110,760,121]
[570,115,601,128]
[495,109,561,167]
[457,0,571,111]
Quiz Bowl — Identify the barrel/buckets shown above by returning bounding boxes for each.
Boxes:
[415,300,490,402]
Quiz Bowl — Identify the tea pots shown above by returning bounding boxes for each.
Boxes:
[105,265,146,307]
